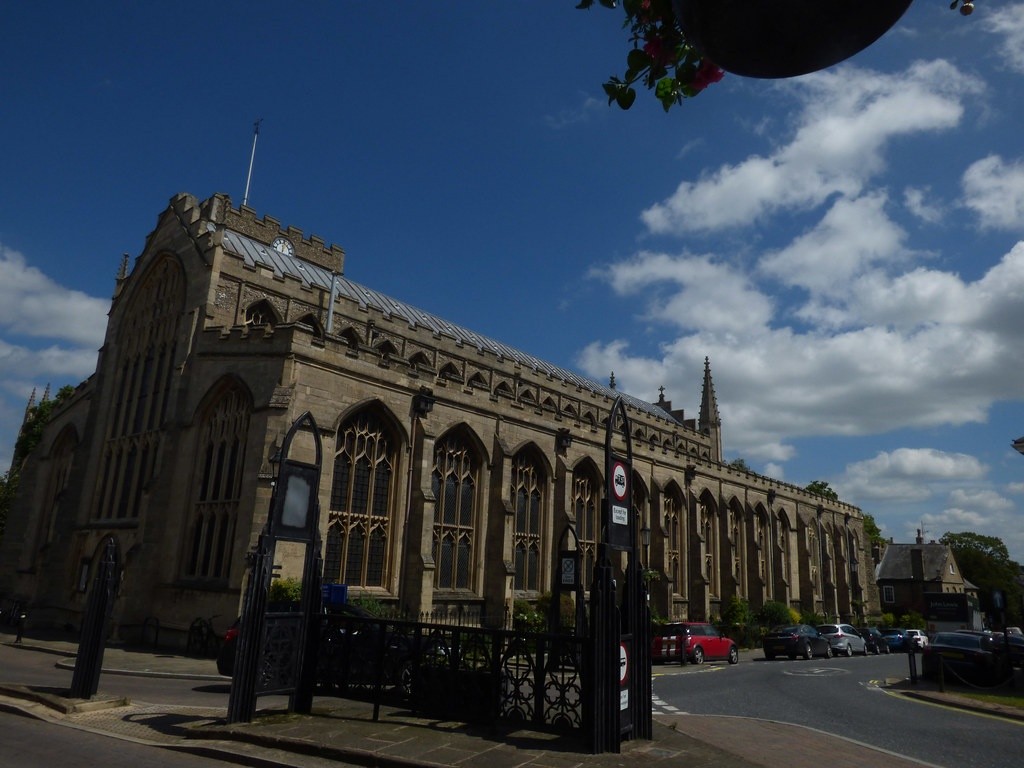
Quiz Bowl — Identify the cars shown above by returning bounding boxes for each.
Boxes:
[922,632,992,679]
[817,625,869,657]
[654,621,738,666]
[217,599,457,697]
[956,627,1024,673]
[761,625,833,661]
[880,629,912,654]
[855,627,890,655]
[904,630,928,648]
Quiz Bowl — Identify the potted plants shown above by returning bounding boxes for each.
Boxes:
[269,577,303,613]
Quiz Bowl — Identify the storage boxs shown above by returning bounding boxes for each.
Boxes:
[321,583,348,605]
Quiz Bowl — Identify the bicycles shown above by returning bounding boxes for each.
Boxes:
[189,614,222,658]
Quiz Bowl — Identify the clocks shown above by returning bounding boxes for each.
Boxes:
[270,237,295,259]
